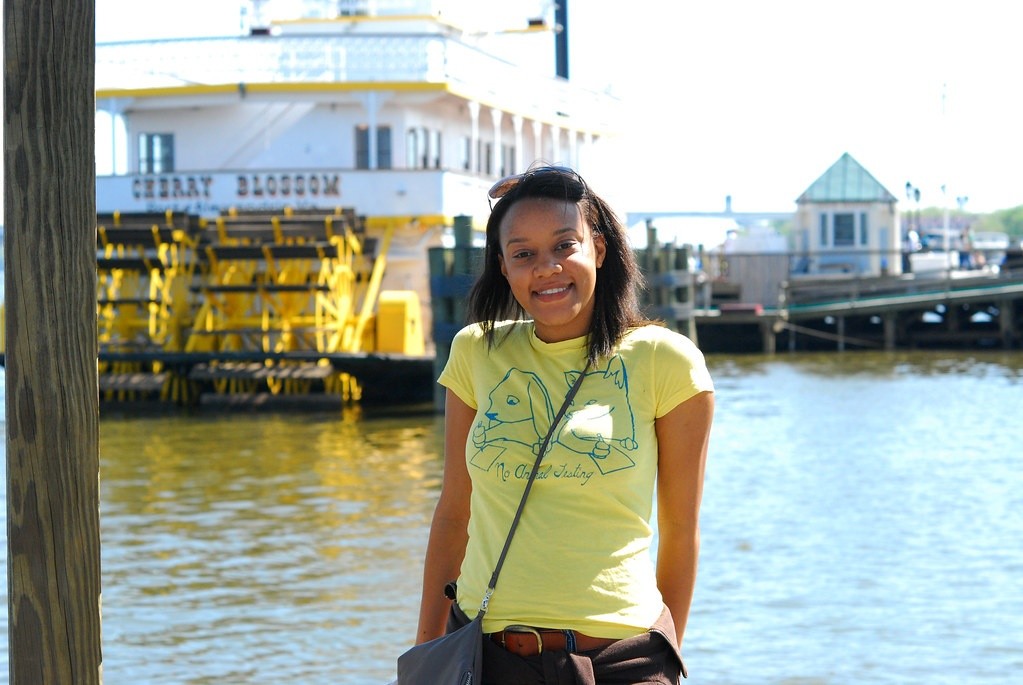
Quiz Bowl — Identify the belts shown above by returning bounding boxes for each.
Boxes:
[485,625,622,657]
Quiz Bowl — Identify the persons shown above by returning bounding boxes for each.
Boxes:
[958,223,984,270]
[903,224,929,254]
[415,163,715,685]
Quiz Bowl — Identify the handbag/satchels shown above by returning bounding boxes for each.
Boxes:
[397,611,483,685]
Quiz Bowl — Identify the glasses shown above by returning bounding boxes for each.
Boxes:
[487,166,591,214]
